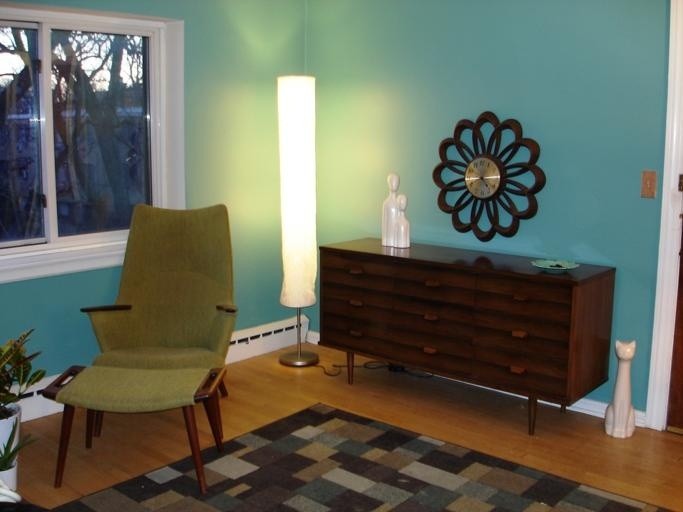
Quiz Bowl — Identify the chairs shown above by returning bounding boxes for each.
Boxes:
[42,204,238,496]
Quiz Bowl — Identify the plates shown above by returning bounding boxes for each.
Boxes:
[529,259,580,275]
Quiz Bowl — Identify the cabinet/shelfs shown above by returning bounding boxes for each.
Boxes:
[318,235,616,435]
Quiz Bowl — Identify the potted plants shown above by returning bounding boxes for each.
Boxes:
[0,416,39,491]
[0,326,46,459]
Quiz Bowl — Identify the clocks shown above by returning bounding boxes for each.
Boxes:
[432,110,547,243]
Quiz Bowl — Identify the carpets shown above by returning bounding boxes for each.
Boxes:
[49,402,679,512]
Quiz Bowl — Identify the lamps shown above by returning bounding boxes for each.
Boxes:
[274,75,320,368]
